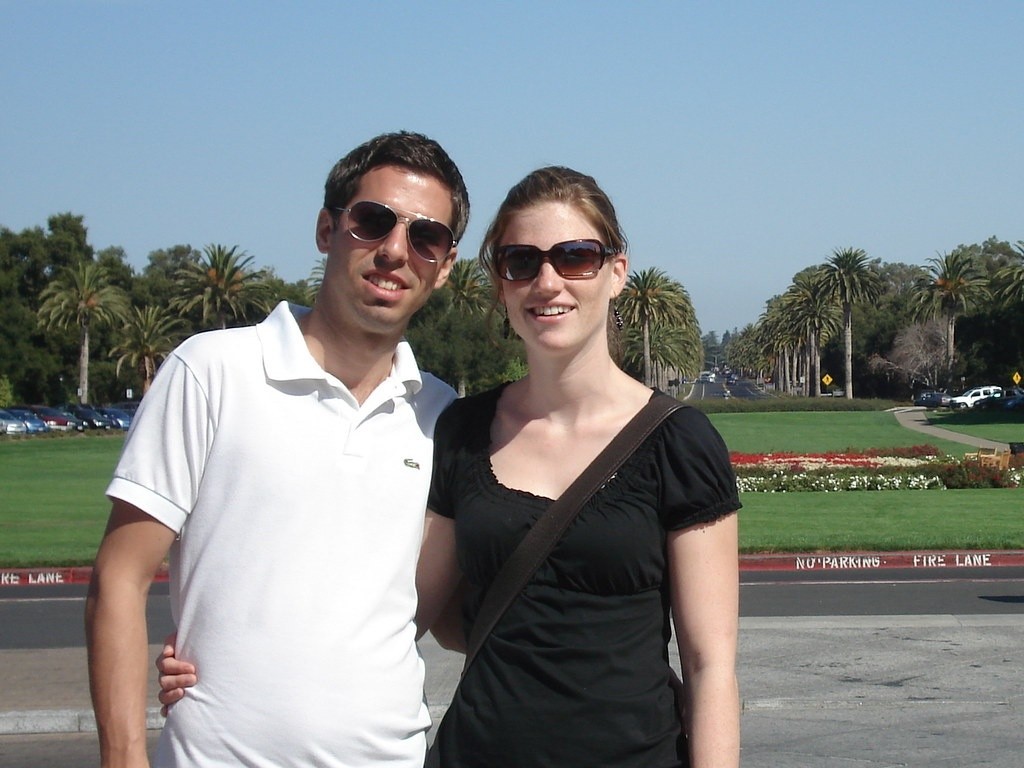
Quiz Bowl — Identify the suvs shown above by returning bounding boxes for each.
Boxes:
[949,385,1005,410]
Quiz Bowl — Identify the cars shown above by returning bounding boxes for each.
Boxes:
[974,388,1024,412]
[913,392,951,407]
[0,401,142,435]
[698,366,738,385]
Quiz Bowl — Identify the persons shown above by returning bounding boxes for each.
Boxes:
[157,166,744,768]
[723,389,731,399]
[84,129,470,768]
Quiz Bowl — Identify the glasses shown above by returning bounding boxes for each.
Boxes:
[333,199,458,263]
[495,239,617,281]
